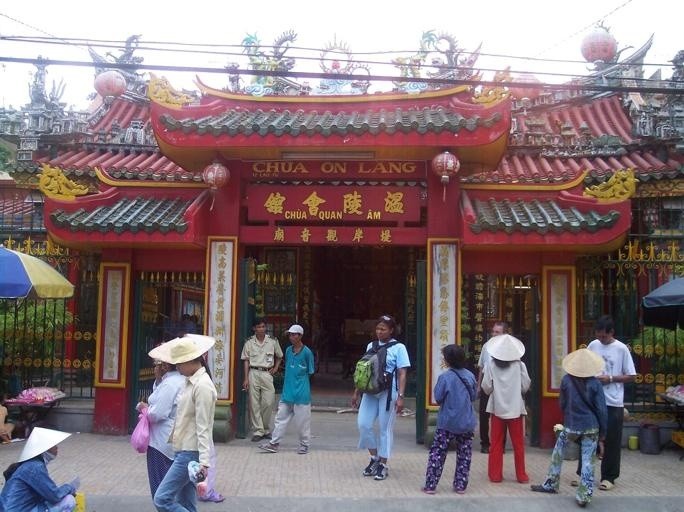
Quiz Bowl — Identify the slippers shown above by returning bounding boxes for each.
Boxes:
[457,490,465,494]
[421,487,436,494]
[598,479,615,490]
[570,475,581,485]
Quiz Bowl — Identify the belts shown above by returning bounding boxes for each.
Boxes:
[249,366,272,372]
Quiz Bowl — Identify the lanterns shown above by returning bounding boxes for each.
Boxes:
[431,151,461,202]
[94,70,126,114]
[581,24,617,65]
[510,75,540,116]
[202,162,231,212]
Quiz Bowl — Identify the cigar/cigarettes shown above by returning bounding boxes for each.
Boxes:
[242,388,245,391]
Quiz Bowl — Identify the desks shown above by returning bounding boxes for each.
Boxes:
[654,391,684,459]
[5,397,69,439]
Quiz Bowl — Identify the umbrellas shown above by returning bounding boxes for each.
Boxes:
[0,244,77,298]
[638,278,684,331]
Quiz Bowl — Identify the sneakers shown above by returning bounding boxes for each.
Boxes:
[259,441,279,452]
[363,457,388,479]
[296,443,308,453]
[251,433,272,443]
[207,491,226,501]
[531,484,556,493]
[576,498,587,505]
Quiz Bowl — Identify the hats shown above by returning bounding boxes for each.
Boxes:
[18,426,72,462]
[285,324,304,334]
[149,332,215,365]
[562,349,605,377]
[486,333,525,360]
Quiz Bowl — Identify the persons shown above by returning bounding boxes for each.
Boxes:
[477,320,531,484]
[257,324,316,455]
[134,312,226,512]
[350,315,412,481]
[240,316,284,442]
[0,426,81,512]
[0,392,16,443]
[571,313,637,491]
[421,344,478,494]
[531,348,609,508]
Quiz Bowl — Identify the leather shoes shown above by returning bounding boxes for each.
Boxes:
[482,444,489,453]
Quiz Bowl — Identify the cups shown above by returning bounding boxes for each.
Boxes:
[628,435,638,450]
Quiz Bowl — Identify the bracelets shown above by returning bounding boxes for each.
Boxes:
[399,393,405,399]
[609,375,612,383]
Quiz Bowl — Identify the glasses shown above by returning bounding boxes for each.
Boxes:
[381,316,390,321]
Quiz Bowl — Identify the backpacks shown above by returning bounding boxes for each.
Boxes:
[354,339,400,395]
